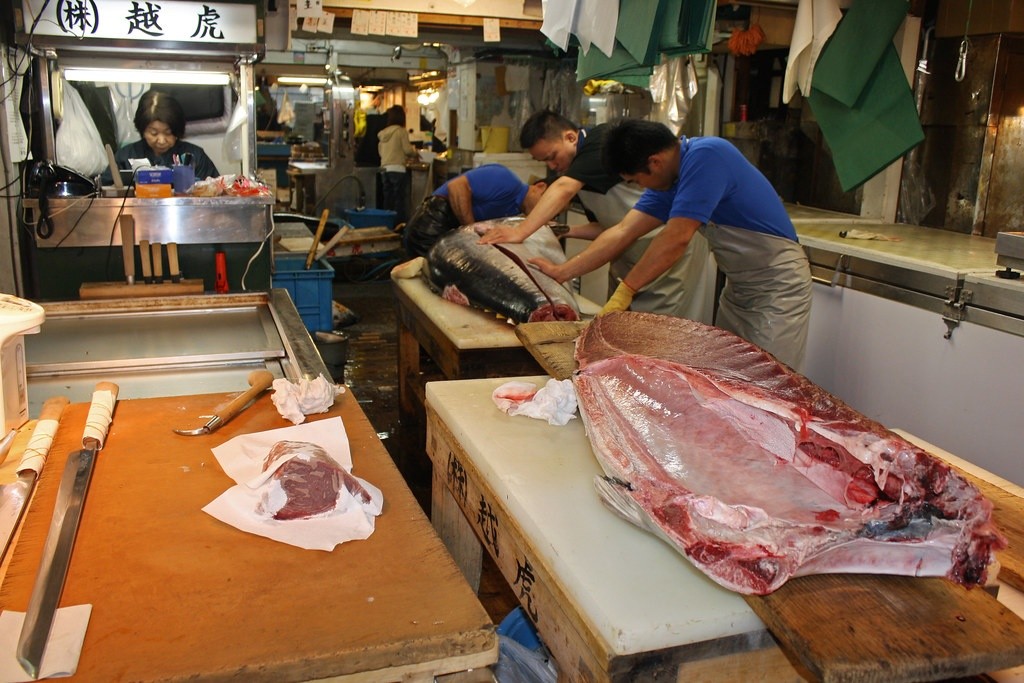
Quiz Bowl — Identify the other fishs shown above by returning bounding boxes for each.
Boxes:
[421,215,587,323]
[575,304,1004,598]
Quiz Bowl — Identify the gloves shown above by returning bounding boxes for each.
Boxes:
[598,281,636,317]
[353,107,366,137]
[583,79,624,95]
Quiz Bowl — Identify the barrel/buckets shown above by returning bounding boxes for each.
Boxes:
[345,208,397,229]
[479,126,509,153]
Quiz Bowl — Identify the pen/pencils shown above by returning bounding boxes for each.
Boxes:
[173,153,181,166]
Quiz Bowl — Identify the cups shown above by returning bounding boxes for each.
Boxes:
[172,164,195,194]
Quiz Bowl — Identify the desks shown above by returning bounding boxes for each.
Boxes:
[286,170,317,213]
[423,375,782,683]
[390,273,605,485]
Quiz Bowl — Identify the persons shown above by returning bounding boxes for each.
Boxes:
[375,100,423,223]
[97,90,219,195]
[394,163,558,261]
[475,104,712,322]
[528,118,814,371]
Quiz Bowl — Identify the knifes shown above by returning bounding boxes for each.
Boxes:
[0,419,59,560]
[17,382,120,679]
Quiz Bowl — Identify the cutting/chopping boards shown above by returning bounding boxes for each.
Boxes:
[516,321,1023,683]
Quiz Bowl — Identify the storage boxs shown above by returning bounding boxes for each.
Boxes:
[275,249,336,334]
[343,207,399,230]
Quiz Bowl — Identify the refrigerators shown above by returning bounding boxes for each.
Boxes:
[792,223,1024,493]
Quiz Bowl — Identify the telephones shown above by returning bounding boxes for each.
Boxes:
[27,160,97,199]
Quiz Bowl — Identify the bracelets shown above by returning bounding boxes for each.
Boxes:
[575,254,584,274]
